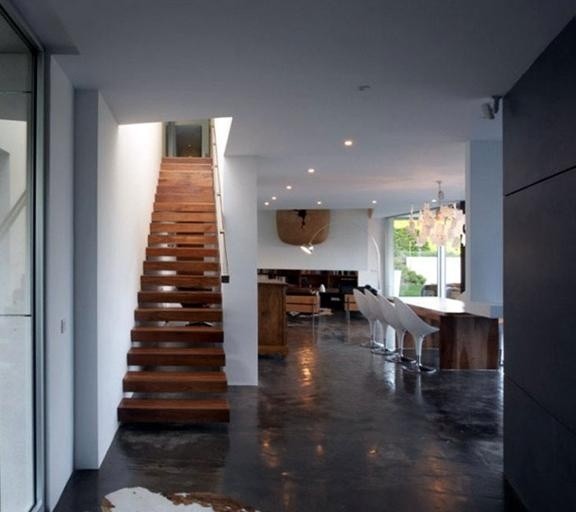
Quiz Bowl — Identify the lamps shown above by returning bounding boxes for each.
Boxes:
[409,180,467,250]
[300,221,381,291]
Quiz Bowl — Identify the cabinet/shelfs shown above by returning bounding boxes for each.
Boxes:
[286,289,344,313]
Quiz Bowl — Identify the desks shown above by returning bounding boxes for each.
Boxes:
[257,280,292,360]
[388,297,499,371]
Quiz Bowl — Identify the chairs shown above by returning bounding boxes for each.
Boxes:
[285,288,321,337]
[345,288,441,374]
